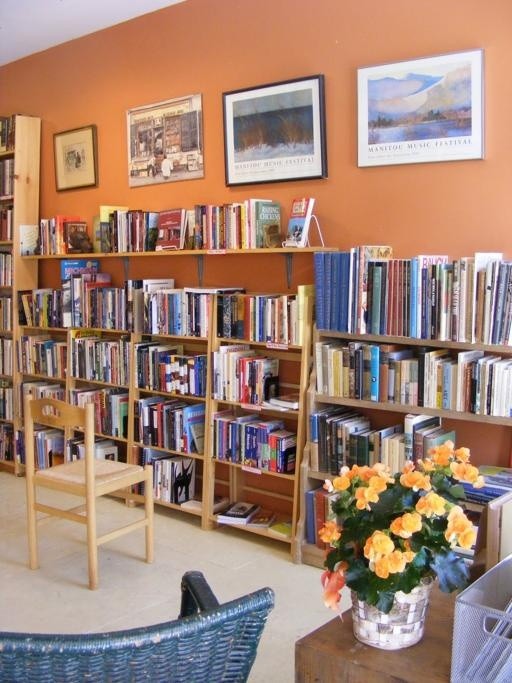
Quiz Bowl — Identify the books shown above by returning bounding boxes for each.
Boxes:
[18,259,143,332]
[143,277,224,337]
[0,206,12,240]
[0,114,22,151]
[21,381,66,418]
[134,396,204,454]
[92,199,280,253]
[315,246,512,346]
[315,340,512,417]
[0,252,12,288]
[0,159,13,196]
[19,224,40,255]
[181,492,229,514]
[19,334,67,378]
[16,424,64,470]
[209,410,297,474]
[463,594,512,683]
[0,381,13,420]
[0,296,12,332]
[134,341,207,398]
[218,285,315,346]
[41,215,88,254]
[219,500,292,538]
[131,446,194,504]
[66,436,118,464]
[69,385,128,438]
[287,197,315,248]
[213,344,299,412]
[309,407,512,505]
[0,338,12,376]
[306,484,346,551]
[0,423,14,461]
[69,329,130,385]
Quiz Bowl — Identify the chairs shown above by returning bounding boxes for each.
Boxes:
[0,570,275,683]
[24,393,154,590]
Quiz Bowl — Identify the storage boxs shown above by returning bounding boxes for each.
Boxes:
[449,554,512,683]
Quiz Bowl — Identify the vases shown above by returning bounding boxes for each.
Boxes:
[351,578,440,652]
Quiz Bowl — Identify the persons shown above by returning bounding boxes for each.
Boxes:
[146,155,156,177]
[161,155,173,181]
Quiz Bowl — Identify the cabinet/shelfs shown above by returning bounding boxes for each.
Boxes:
[290,324,512,576]
[294,581,461,683]
[0,114,41,476]
[16,245,339,542]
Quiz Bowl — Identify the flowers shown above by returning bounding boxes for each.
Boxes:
[317,438,485,623]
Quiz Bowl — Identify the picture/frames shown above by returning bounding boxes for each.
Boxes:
[222,74,329,187]
[357,47,485,167]
[126,94,204,188]
[52,123,100,192]
